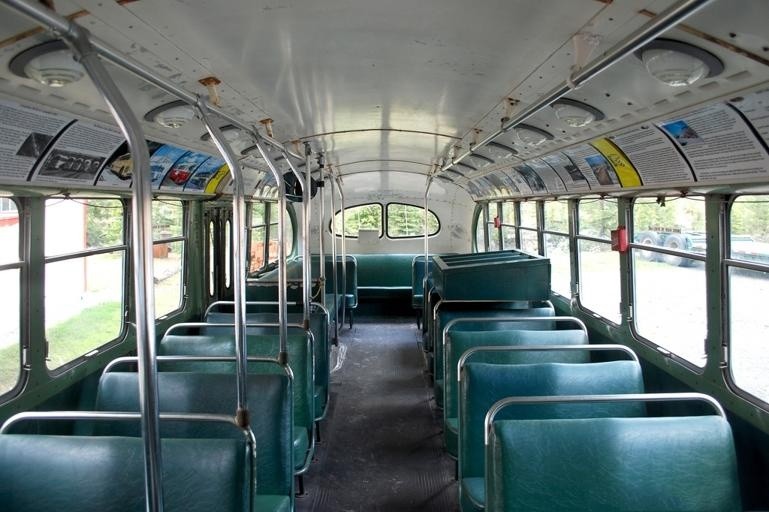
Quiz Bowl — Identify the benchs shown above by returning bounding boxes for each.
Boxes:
[93,356,295,512]
[158,323,316,495]
[442,316,591,480]
[1,412,256,512]
[434,300,556,409]
[486,393,742,512]
[428,286,440,355]
[294,255,357,328]
[422,272,434,336]
[460,344,648,512]
[412,253,461,329]
[246,279,341,347]
[199,301,330,442]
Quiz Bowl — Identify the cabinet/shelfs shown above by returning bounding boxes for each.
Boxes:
[432,248,552,301]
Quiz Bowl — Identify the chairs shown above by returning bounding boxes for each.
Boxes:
[352,253,414,290]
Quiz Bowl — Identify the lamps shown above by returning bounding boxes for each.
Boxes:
[513,123,554,147]
[445,170,464,179]
[9,39,87,88]
[311,167,320,180]
[485,141,518,160]
[456,162,477,177]
[144,99,194,129]
[200,124,241,147]
[548,97,605,129]
[469,154,495,169]
[634,38,727,89]
[437,175,454,184]
[289,160,307,177]
[324,176,330,182]
[241,142,272,158]
[275,156,286,164]
